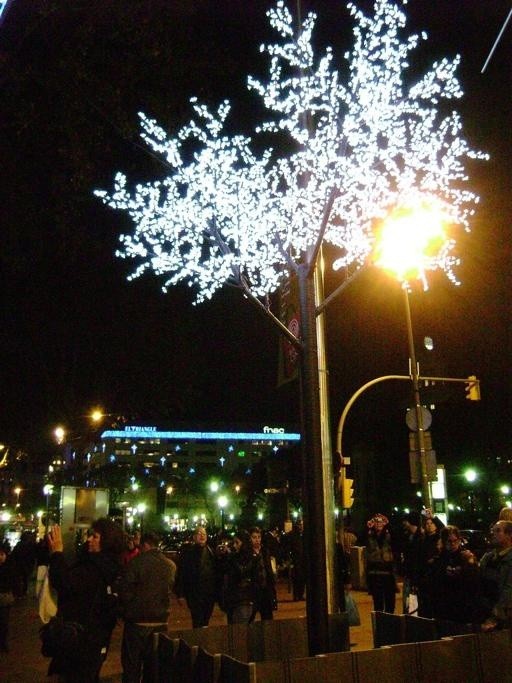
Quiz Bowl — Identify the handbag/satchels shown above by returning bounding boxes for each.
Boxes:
[228,571,262,605]
[41,621,85,658]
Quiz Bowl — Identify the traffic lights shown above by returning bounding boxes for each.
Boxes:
[343,479,354,508]
[464,375,481,401]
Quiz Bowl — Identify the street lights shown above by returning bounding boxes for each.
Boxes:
[465,470,476,529]
[135,500,149,549]
[43,484,54,535]
[217,496,228,531]
[374,202,448,510]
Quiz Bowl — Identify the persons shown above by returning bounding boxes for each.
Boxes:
[0,507,512,683]
